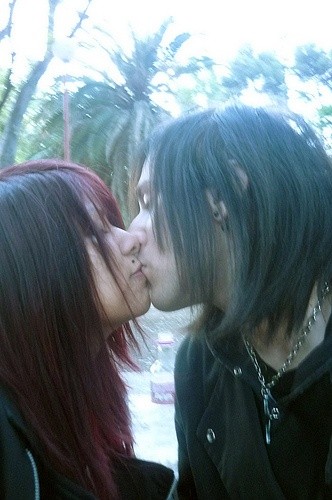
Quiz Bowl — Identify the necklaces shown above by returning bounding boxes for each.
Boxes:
[239,277,330,444]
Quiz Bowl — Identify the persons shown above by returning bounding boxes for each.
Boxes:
[126,100,332,500]
[0,158,177,499]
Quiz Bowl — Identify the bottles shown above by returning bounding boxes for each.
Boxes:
[148,331,178,446]
